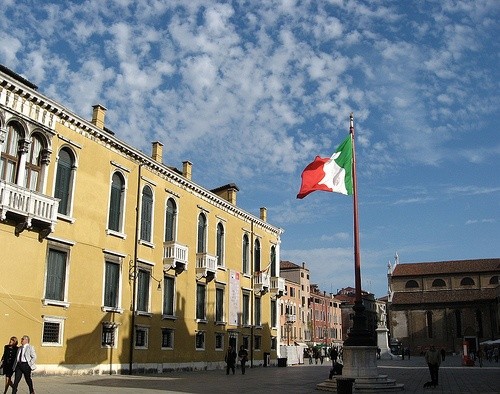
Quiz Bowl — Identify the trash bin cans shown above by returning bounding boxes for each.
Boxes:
[277,357,287,367]
[336,378,356,394]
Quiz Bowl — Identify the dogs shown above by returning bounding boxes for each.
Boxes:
[423,381,432,389]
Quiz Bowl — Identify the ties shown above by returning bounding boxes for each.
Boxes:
[19,347,22,363]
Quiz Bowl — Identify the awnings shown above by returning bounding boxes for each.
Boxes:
[297,343,309,348]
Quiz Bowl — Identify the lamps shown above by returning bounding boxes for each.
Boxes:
[195,271,215,285]
[254,287,268,297]
[275,291,283,300]
[163,261,185,277]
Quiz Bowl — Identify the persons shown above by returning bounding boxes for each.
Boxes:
[307,345,344,381]
[377,344,500,386]
[238,346,248,375]
[0,335,36,394]
[225,346,237,375]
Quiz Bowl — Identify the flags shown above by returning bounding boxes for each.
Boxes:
[297,132,354,199]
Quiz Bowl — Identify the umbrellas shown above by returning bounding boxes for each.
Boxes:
[479,340,500,354]
